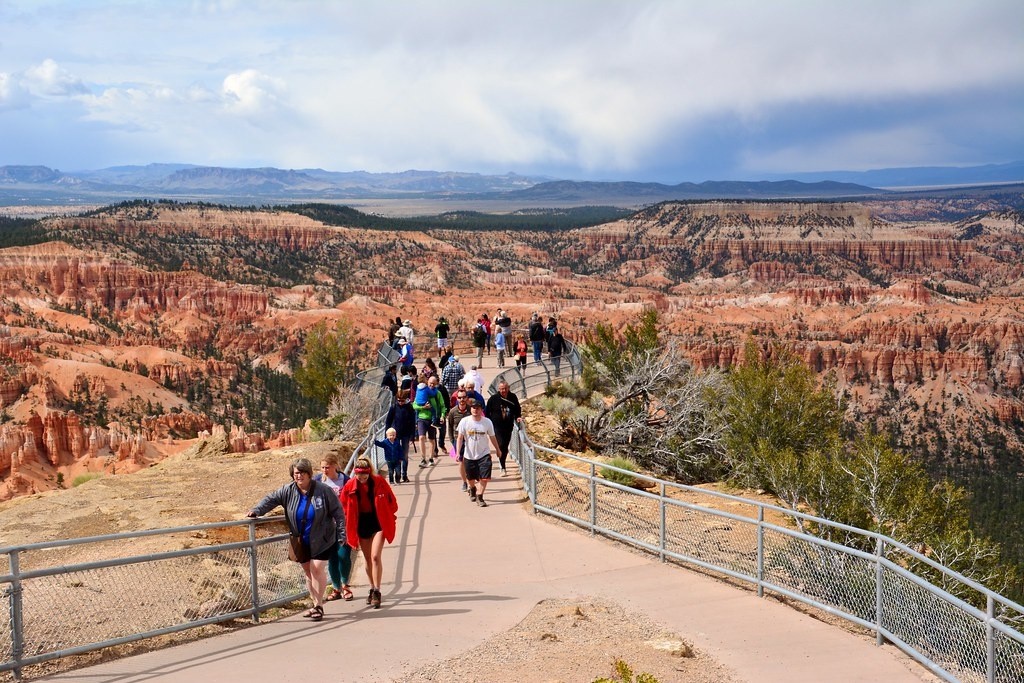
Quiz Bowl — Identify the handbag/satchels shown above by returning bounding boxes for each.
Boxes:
[514,355,520,360]
[288,536,302,562]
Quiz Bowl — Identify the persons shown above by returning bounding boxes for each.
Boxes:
[372,309,568,507]
[243,452,398,620]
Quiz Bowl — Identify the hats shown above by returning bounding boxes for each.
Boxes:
[398,339,407,344]
[403,320,411,325]
[395,331,403,336]
[470,401,482,407]
[448,357,455,362]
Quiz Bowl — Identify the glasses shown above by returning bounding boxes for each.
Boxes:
[398,400,405,402]
[522,337,524,338]
[458,396,466,399]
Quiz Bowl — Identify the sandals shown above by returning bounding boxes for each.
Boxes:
[342,587,353,601]
[311,606,324,620]
[303,610,312,617]
[326,589,341,601]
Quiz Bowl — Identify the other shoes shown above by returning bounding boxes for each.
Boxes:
[429,459,434,466]
[434,453,438,457]
[366,589,374,604]
[431,423,441,428]
[462,482,467,491]
[371,591,381,608]
[442,446,447,453]
[470,489,476,501]
[500,469,506,476]
[402,479,409,482]
[419,460,427,467]
[476,495,486,507]
